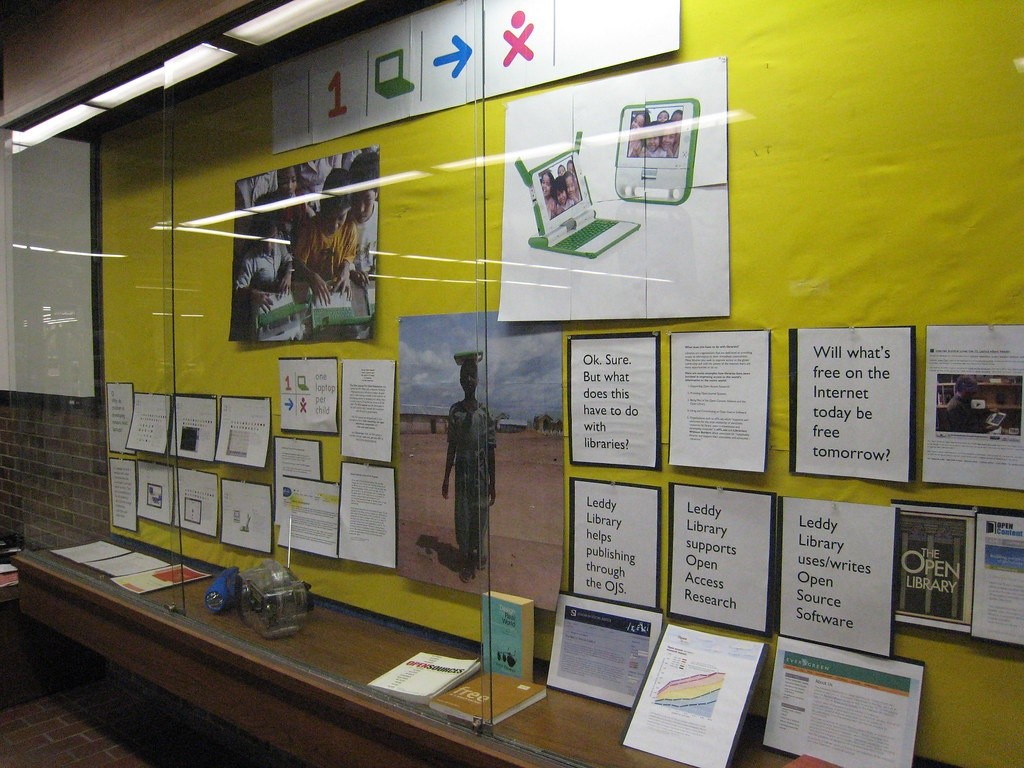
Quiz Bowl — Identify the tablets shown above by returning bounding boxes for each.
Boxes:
[614,98,700,205]
[988,411,1006,427]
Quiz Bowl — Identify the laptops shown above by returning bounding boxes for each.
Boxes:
[257,291,375,337]
[515,130,641,259]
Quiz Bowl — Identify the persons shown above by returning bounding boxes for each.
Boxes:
[628,110,683,157]
[228,152,379,314]
[543,160,582,219]
[442,360,497,583]
[947,375,998,434]
